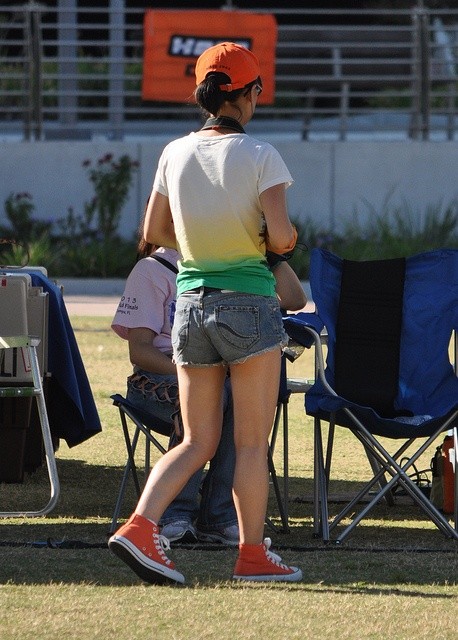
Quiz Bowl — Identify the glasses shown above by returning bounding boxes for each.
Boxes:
[244,80,262,96]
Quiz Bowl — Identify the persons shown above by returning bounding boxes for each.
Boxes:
[110,196,308,546]
[108,41,304,584]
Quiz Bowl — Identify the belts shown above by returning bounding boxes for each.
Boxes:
[183,287,223,294]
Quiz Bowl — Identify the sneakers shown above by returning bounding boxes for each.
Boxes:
[233,537,302,581]
[158,520,199,543]
[108,514,185,585]
[195,525,239,547]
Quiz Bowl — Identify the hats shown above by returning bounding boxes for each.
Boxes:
[195,42,263,92]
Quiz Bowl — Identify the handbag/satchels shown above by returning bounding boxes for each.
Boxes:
[430,436,454,513]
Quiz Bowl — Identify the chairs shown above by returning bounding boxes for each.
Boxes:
[288,248,457,547]
[109,303,291,542]
